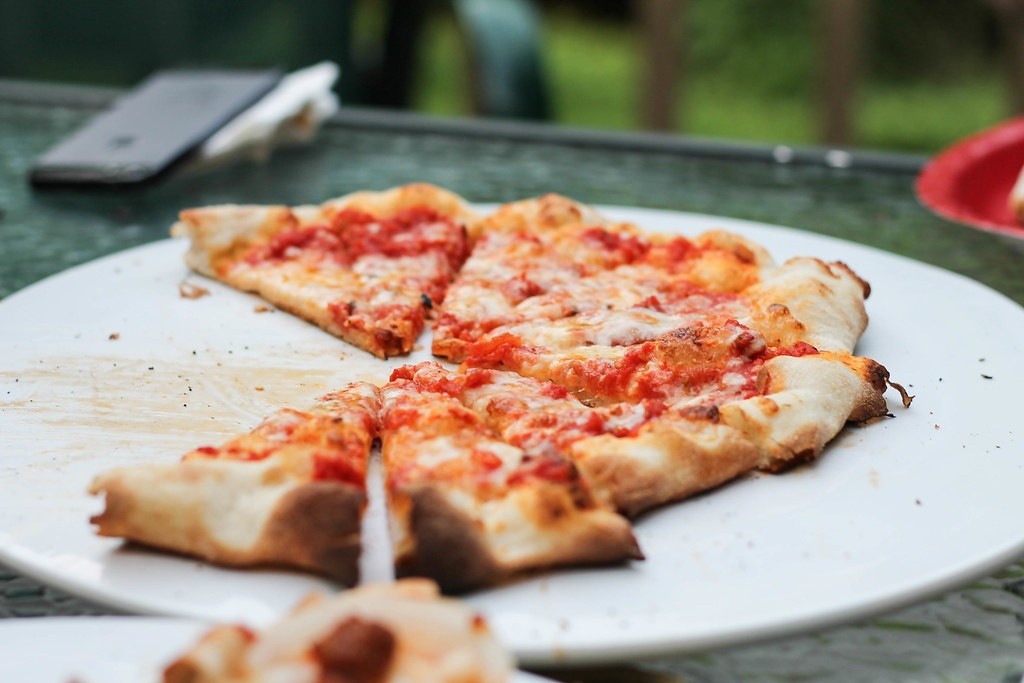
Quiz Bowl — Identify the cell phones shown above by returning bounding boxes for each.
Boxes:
[29,69,283,195]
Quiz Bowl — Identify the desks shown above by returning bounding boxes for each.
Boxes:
[0,82,1024,683]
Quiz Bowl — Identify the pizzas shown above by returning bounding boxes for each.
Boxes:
[87,183,913,598]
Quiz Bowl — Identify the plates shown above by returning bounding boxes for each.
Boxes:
[917,117,1024,252]
[0,202,1024,666]
[0,617,560,683]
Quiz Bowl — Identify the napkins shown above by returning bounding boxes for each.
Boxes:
[180,59,341,177]
